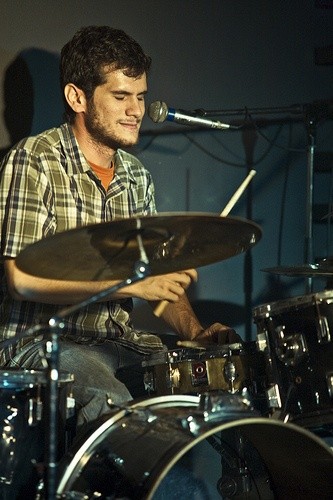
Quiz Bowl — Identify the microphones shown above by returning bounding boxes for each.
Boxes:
[147,101,239,131]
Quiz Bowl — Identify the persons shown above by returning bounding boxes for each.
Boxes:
[0,26,244,451]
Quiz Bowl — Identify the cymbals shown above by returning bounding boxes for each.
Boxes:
[12,210,265,282]
[256,262,333,279]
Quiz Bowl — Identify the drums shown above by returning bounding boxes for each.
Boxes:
[140,342,255,399]
[251,287,333,434]
[1,369,78,500]
[54,393,332,500]
